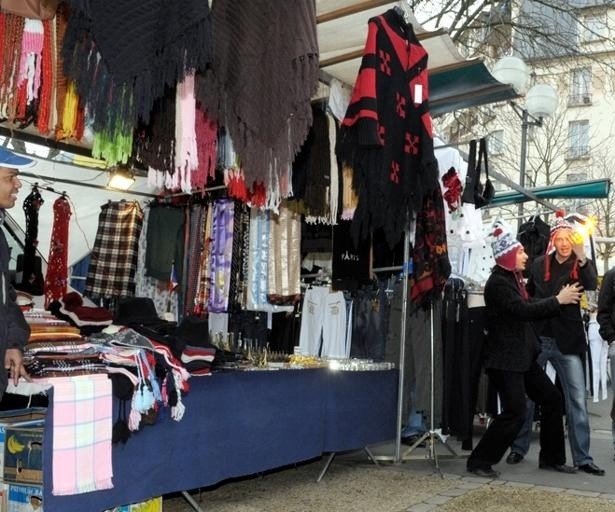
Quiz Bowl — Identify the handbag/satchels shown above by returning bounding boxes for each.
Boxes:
[463,174,497,205]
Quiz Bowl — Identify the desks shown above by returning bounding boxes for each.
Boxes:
[1,359,400,512]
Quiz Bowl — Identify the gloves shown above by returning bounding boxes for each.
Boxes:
[608,340,615,360]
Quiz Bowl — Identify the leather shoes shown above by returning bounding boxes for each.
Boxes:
[539,462,605,476]
[467,458,501,478]
[506,448,523,465]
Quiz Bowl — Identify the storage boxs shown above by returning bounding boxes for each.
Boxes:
[1,407,163,512]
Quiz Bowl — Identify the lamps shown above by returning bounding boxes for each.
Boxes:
[105,168,135,192]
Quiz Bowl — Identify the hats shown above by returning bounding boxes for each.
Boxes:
[491,228,524,272]
[549,209,574,240]
[0,140,34,168]
[90,298,185,387]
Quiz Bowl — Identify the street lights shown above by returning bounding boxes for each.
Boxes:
[491,55,560,242]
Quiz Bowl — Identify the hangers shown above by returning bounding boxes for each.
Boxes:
[145,185,230,206]
[390,1,409,31]
[27,182,40,195]
[318,70,333,115]
[584,307,599,315]
[60,191,68,201]
[300,271,466,295]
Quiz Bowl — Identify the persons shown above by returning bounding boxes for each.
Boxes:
[0,146,41,400]
[466,225,586,480]
[501,216,608,479]
[596,264,615,460]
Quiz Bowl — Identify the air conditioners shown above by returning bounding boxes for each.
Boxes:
[579,93,593,105]
